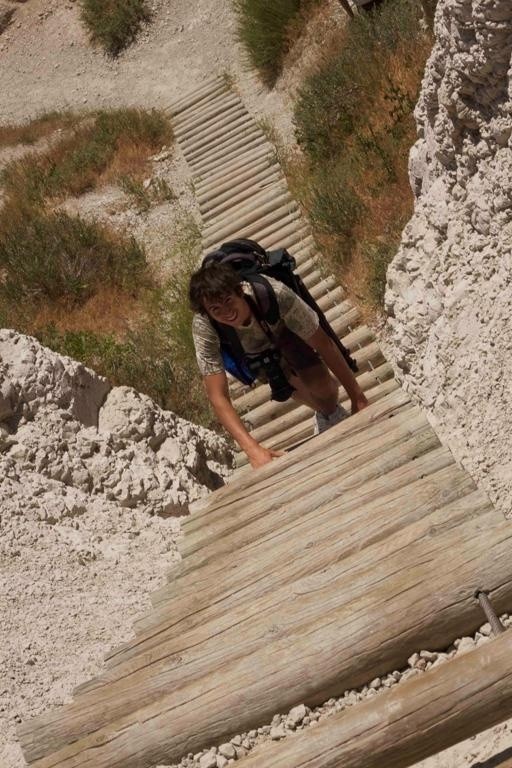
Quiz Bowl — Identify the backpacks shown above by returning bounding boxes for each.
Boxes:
[202,238,302,355]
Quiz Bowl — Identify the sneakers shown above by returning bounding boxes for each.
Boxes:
[313,402,351,435]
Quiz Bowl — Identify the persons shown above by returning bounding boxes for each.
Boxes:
[187,259,370,471]
